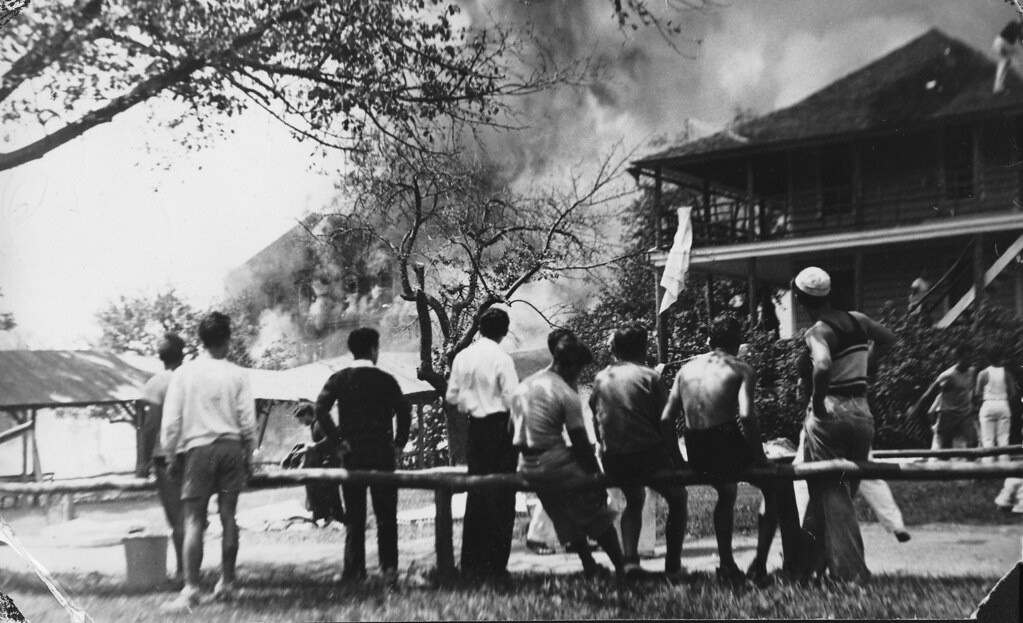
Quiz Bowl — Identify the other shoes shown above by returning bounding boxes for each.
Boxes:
[162,584,231,609]
[718,560,766,583]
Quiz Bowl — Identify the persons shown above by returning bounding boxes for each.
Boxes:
[314,328,412,583]
[446,308,519,584]
[905,344,981,462]
[974,346,1017,461]
[282,398,345,528]
[859,479,911,542]
[133,335,194,587]
[160,312,259,608]
[510,328,627,585]
[990,20,1023,96]
[792,266,897,581]
[588,323,696,586]
[659,314,779,583]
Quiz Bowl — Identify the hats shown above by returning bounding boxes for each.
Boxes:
[790,267,832,300]
